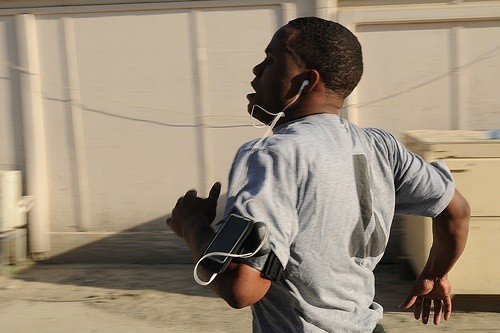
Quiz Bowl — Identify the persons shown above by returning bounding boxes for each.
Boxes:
[166,19,471,333]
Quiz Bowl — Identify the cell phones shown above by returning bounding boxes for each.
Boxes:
[199,213,254,275]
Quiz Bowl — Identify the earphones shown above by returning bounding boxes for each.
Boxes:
[298,79,310,91]
